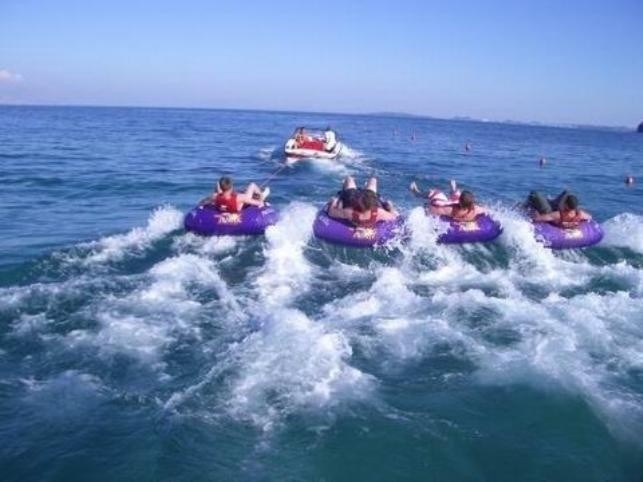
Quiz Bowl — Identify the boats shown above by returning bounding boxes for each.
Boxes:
[508,202,605,248]
[311,196,404,245]
[284,126,345,160]
[180,195,279,235]
[423,204,500,242]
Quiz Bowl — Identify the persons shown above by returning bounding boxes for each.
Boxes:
[322,126,337,153]
[327,175,396,227]
[522,188,591,228]
[211,176,271,215]
[409,180,486,222]
[293,127,309,149]
